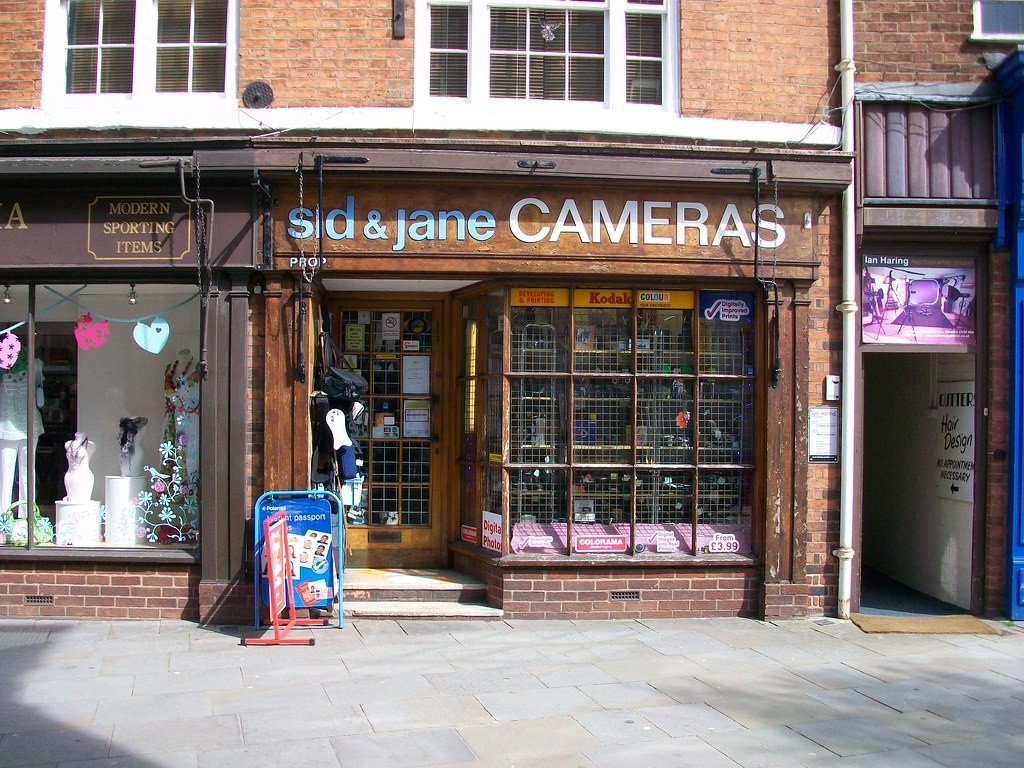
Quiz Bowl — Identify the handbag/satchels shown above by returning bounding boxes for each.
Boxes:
[0,500,53,544]
[310,367,368,485]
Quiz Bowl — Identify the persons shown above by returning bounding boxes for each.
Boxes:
[0,344,44,543]
[155,347,202,534]
[119,416,147,478]
[64,431,98,502]
[285,533,328,599]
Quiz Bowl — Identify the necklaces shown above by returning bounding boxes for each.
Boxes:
[170,356,193,390]
[66,437,89,470]
